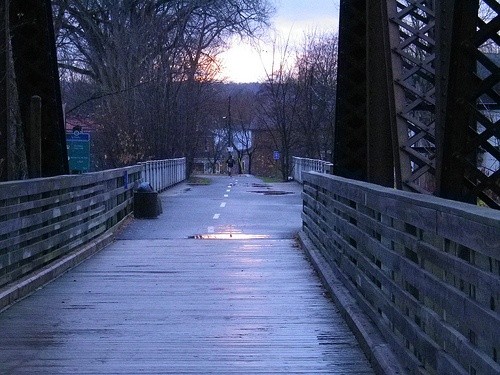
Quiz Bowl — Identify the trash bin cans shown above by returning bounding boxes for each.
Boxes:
[134,190,159,219]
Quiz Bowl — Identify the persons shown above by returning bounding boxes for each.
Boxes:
[226,155,236,175]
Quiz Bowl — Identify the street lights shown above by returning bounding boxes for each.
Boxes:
[222,96,233,157]
